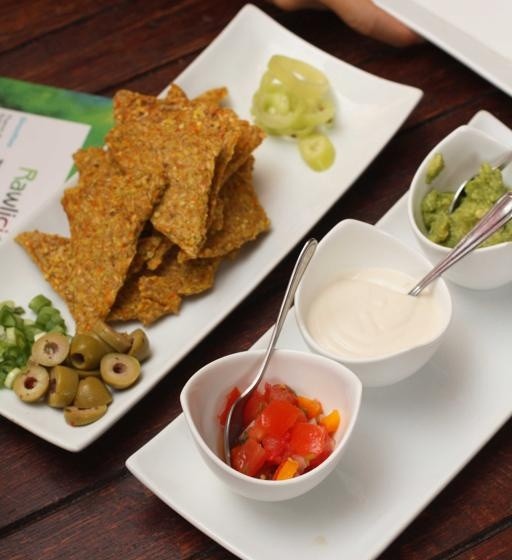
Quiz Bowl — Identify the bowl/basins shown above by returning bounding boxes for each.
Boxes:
[291,216,457,390]
[177,345,367,505]
[406,120,512,290]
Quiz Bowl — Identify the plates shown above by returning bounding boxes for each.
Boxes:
[117,107,511,560]
[1,2,426,451]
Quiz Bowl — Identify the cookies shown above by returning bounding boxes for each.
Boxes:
[13,83,270,337]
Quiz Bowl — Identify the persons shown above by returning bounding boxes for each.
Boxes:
[272,1,427,49]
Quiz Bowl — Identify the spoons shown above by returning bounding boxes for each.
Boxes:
[449,149,512,218]
[221,233,319,469]
